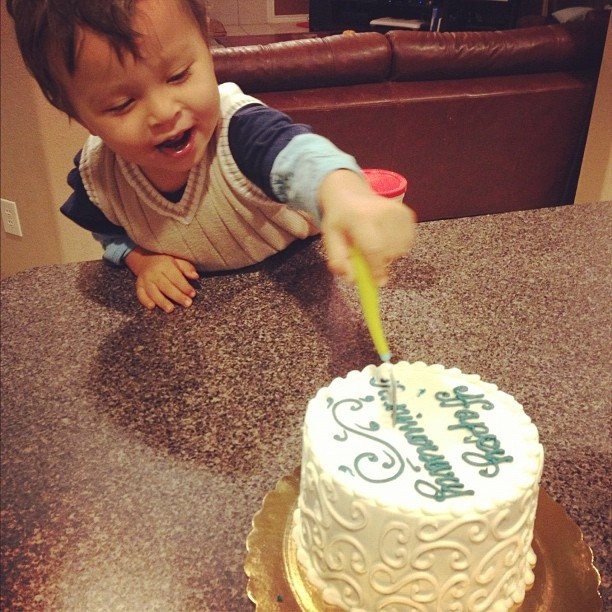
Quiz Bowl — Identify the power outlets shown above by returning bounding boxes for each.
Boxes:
[1,198,22,237]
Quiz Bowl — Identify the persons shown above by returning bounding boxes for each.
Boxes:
[5,1,416,313]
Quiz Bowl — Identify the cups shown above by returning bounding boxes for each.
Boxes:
[360,168,408,288]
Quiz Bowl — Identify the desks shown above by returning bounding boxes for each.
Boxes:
[0,200,611,611]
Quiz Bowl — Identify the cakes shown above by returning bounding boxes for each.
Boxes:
[291,361,544,611]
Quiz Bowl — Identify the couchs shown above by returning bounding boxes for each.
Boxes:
[211,13,591,221]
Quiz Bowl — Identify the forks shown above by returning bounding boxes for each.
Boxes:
[352,251,397,428]
